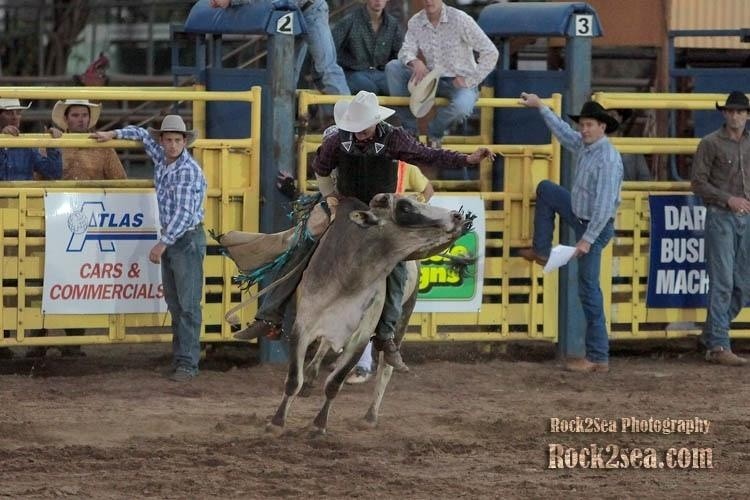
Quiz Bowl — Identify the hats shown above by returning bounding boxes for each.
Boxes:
[568,102,618,133]
[148,115,197,147]
[334,91,396,133]
[52,100,103,130]
[716,91,749,111]
[0,98,33,110]
[408,67,441,118]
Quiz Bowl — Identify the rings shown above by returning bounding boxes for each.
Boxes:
[739,208,743,213]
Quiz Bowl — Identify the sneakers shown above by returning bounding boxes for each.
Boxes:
[564,357,609,372]
[519,248,548,265]
[373,338,409,373]
[347,367,371,384]
[155,363,198,382]
[233,319,282,340]
[700,336,746,365]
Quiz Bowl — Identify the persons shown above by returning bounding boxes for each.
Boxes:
[517,90,626,375]
[327,160,435,387]
[208,0,351,119]
[0,97,63,357]
[384,1,500,148]
[605,108,653,182]
[25,98,128,359]
[232,89,497,373]
[86,111,208,383]
[302,0,425,132]
[690,90,750,366]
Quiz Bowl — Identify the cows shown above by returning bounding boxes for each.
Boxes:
[265,191,480,440]
